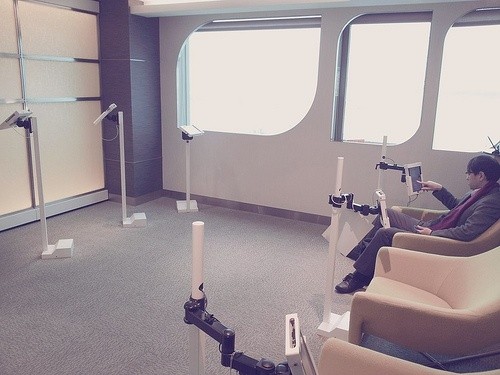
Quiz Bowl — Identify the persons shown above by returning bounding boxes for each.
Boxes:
[335,155,500,293]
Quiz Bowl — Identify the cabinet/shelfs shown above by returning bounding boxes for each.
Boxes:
[1,1,109,233]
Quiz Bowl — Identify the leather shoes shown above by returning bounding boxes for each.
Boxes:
[335,273,365,292]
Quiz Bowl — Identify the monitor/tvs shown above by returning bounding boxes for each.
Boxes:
[286,313,316,375]
[404,163,423,196]
[376,191,391,229]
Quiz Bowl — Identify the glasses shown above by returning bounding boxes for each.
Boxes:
[465,172,473,174]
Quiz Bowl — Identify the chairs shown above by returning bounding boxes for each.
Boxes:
[318,337,500,375]
[349,247,499,356]
[389,205,500,257]
